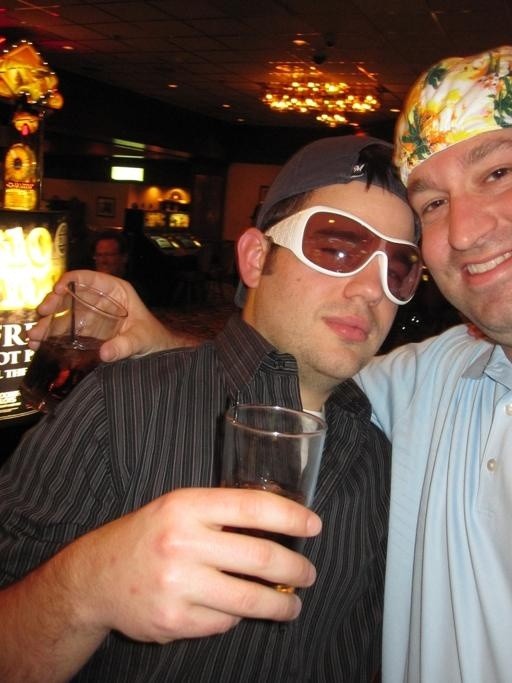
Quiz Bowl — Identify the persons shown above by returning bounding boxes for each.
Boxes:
[27,46,511,683]
[91,229,126,279]
[1,134,424,682]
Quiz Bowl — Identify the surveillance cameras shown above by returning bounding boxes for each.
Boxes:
[312,52,326,64]
[324,32,336,47]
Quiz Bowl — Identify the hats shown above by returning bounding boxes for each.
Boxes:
[392,45,512,189]
[233,135,422,310]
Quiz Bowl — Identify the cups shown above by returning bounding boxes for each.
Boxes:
[19,282,128,412]
[222,401,327,595]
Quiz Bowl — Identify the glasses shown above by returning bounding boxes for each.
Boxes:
[264,205,422,305]
